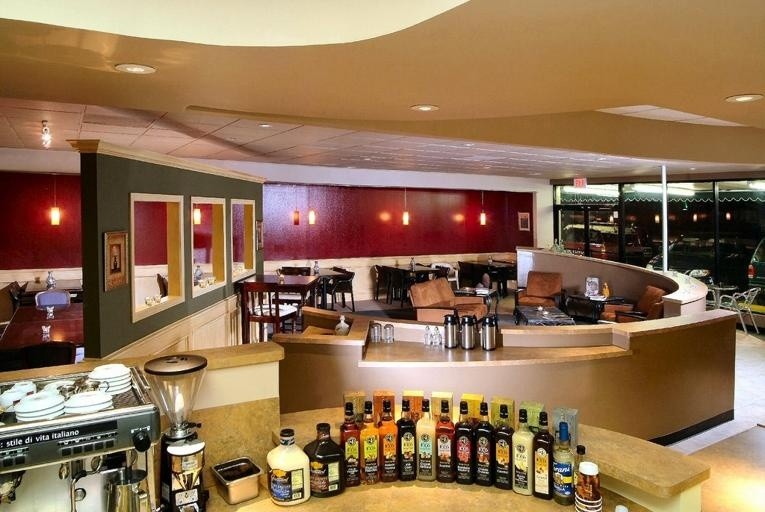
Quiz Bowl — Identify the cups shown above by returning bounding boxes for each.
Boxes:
[574,460,603,511]
[370,323,394,344]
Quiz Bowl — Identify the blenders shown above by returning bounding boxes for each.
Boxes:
[0,367,158,511]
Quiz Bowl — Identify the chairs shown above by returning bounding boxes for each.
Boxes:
[0,278,84,373]
[155,271,168,298]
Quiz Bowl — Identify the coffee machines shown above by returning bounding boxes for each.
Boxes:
[143,353,209,512]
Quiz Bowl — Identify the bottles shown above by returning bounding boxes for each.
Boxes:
[602,282,610,298]
[336,314,349,335]
[424,325,442,347]
[410,257,416,268]
[488,255,492,265]
[194,265,203,281]
[266,427,309,507]
[339,397,576,507]
[313,260,320,275]
[303,422,346,499]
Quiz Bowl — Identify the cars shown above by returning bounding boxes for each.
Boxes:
[640,241,653,259]
[645,231,746,282]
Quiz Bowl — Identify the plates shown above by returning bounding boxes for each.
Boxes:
[42,379,78,396]
[86,362,135,396]
[63,390,118,416]
[14,391,67,423]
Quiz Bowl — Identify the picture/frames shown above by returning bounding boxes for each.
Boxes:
[255,219,265,251]
[104,229,130,292]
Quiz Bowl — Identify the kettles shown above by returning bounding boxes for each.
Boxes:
[103,469,153,512]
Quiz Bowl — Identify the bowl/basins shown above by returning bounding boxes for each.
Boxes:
[0,379,36,411]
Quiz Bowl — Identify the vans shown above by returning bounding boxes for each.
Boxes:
[562,223,643,265]
[748,237,765,294]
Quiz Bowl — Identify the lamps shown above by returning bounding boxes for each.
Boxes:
[307,184,315,225]
[402,188,410,226]
[480,191,486,225]
[50,176,61,226]
[193,204,201,224]
[41,119,52,150]
